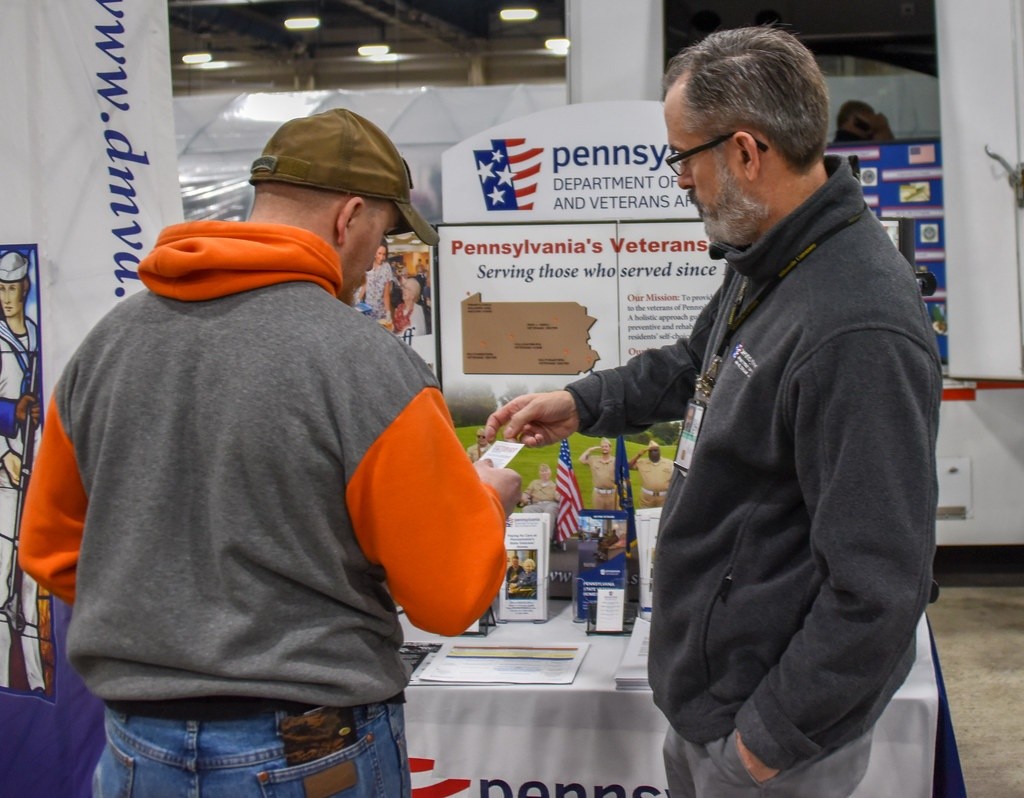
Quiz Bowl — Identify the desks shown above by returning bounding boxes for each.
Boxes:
[391,506,970,797]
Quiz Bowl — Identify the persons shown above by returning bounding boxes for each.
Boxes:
[486,27,942,798]
[521,464,556,504]
[466,428,492,463]
[356,238,431,336]
[506,556,537,599]
[834,100,893,143]
[627,440,675,510]
[580,437,621,510]
[16,107,521,798]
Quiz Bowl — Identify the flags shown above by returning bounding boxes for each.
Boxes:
[556,438,585,551]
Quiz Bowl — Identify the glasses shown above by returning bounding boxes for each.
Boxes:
[665,131,769,176]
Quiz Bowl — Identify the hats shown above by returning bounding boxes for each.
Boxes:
[248,108,440,246]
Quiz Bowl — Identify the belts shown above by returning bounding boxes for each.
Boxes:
[104,689,407,723]
